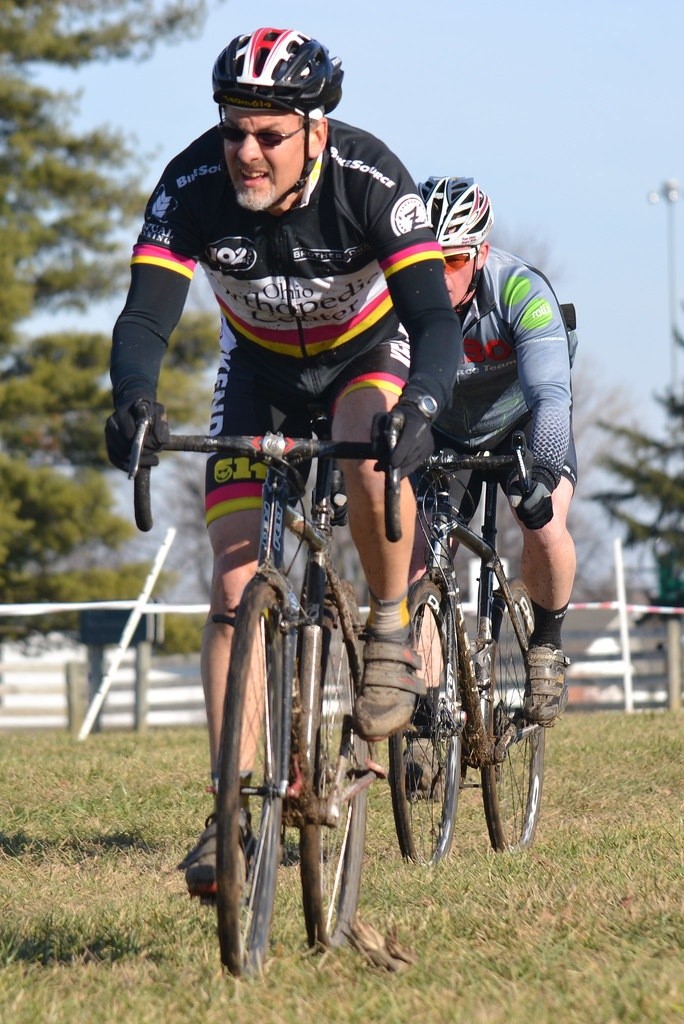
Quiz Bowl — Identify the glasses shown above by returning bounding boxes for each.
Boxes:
[217,118,312,147]
[444,243,483,276]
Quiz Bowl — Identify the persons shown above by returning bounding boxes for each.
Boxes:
[386,173,583,802]
[103,28,464,898]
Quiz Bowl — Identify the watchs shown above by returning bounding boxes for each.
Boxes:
[399,392,442,423]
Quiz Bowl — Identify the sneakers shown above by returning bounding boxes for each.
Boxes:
[522,642,570,725]
[387,752,443,802]
[176,809,258,896]
[352,616,426,743]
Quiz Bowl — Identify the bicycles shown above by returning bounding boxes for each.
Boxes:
[387,445,550,862]
[126,396,405,981]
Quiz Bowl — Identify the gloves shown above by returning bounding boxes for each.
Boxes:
[106,393,167,472]
[509,473,555,531]
[370,404,435,481]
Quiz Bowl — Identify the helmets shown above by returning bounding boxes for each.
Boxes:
[416,177,495,247]
[213,28,342,119]
